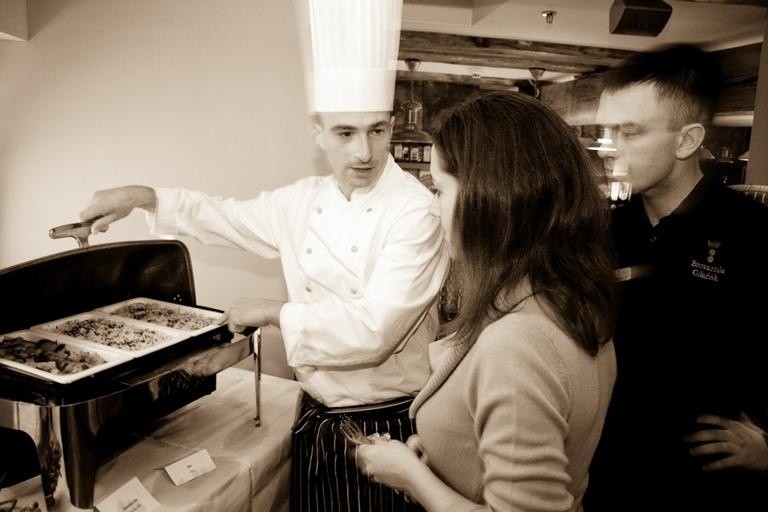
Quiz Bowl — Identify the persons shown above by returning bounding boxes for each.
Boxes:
[351,91,617,512]
[79,111,451,512]
[595,43,768,512]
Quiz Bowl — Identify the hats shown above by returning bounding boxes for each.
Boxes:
[306,1,402,114]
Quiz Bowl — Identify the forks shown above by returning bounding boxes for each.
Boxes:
[340,415,372,444]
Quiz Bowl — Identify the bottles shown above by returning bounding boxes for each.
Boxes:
[714,147,736,185]
